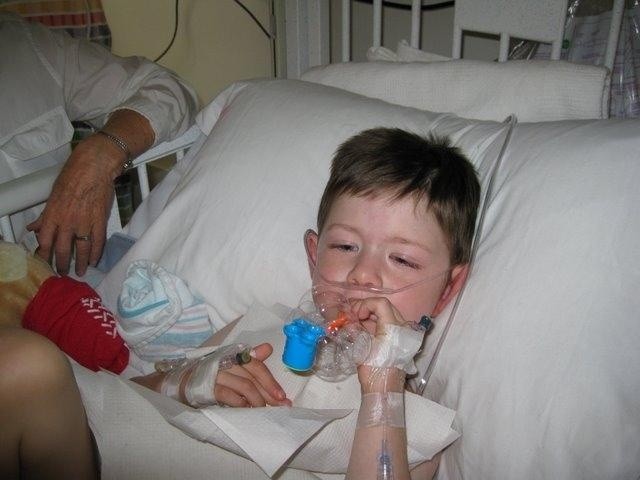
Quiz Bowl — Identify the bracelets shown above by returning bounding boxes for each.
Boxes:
[96,130,132,170]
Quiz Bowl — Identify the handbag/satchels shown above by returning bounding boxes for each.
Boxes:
[508,1,640,117]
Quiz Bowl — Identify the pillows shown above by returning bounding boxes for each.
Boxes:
[94,74,640,480]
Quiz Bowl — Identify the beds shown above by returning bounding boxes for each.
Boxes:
[1,0,640,480]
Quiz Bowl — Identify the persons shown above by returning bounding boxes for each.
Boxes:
[0,9,202,277]
[1,123,482,479]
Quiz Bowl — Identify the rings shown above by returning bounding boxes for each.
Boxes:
[74,236,91,242]
